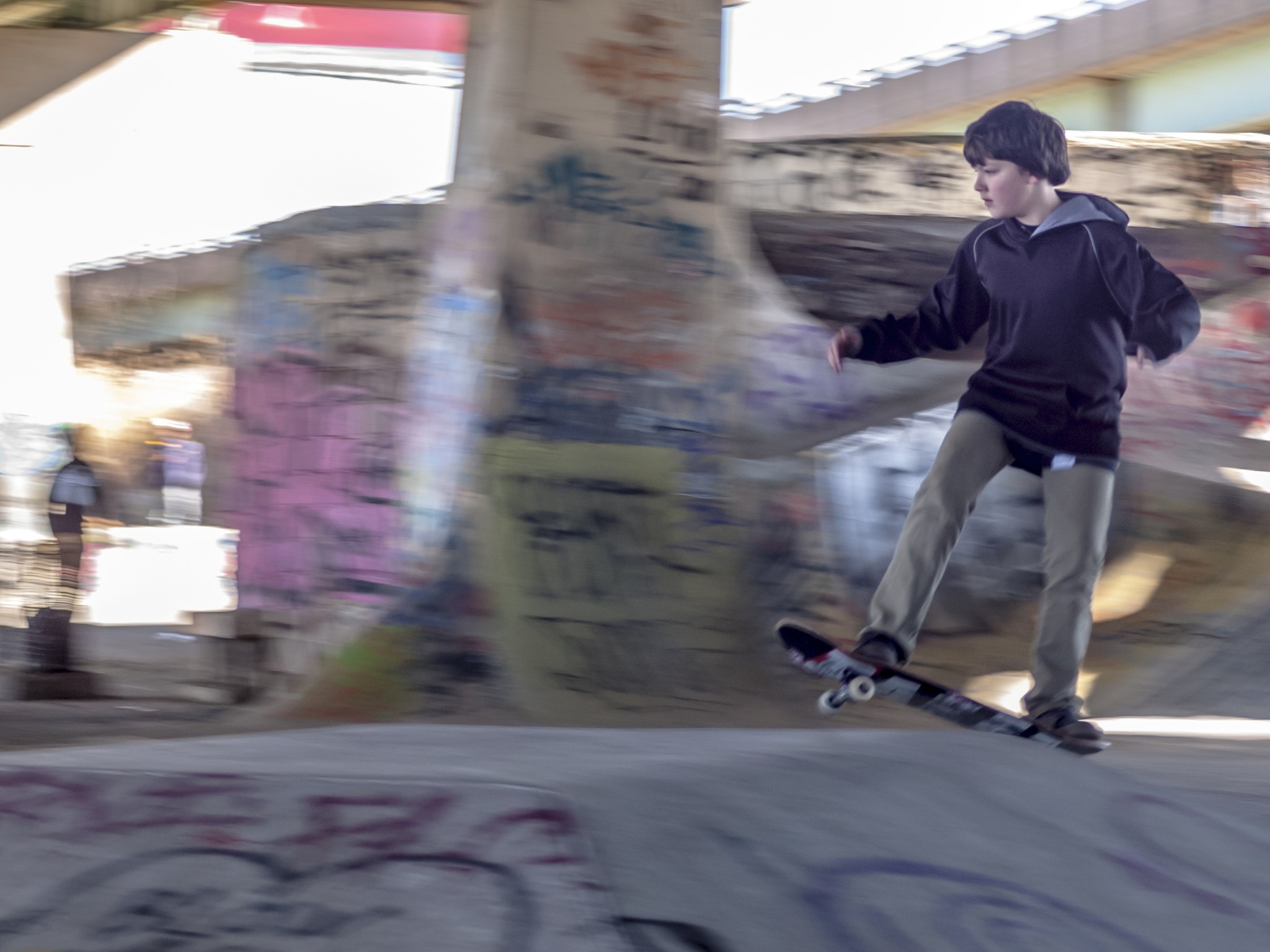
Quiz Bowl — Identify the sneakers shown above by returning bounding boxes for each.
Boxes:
[850,631,911,675]
[1023,702,1114,761]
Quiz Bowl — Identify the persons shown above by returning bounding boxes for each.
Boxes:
[48,425,117,612]
[823,99,1204,744]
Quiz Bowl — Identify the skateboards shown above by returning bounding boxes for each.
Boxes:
[775,618,1111,761]
